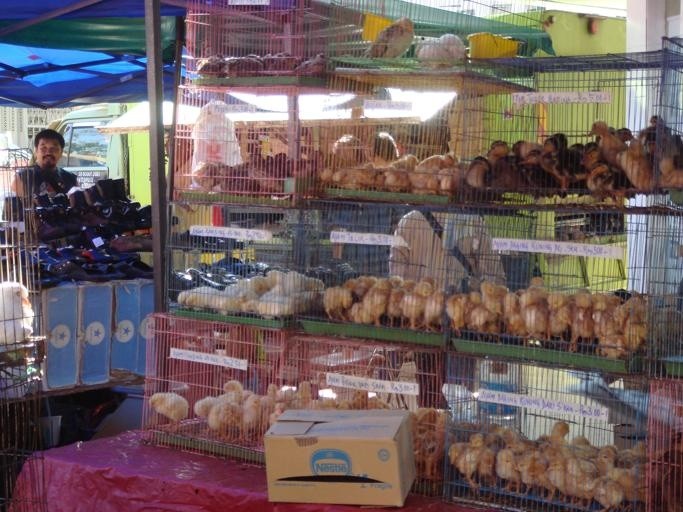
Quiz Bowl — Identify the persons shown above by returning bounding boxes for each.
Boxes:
[370,209,510,409]
[329,134,403,280]
[598,187,682,386]
[326,135,367,259]
[9,128,81,196]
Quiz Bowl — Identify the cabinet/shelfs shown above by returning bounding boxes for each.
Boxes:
[152,1,683,509]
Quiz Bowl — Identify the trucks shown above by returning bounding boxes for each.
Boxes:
[28,9,625,294]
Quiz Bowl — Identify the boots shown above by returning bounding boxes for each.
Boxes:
[0,176,153,290]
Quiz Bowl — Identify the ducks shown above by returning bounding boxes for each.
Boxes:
[194,114,683,195]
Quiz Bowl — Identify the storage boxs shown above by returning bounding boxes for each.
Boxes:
[261,409,416,506]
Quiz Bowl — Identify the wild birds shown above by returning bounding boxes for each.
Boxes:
[367,16,415,58]
[0,281,34,346]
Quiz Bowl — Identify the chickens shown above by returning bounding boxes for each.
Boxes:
[175,268,682,356]
[145,380,682,509]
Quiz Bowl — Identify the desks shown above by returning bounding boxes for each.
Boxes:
[28,427,458,509]
